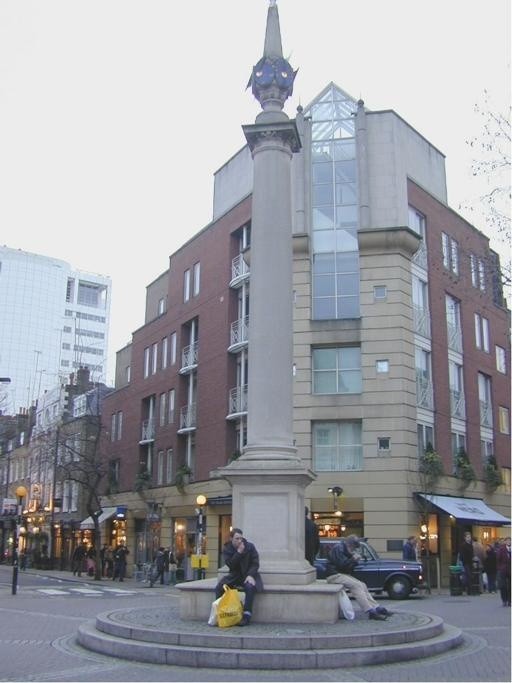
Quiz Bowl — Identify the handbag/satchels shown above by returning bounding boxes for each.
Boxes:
[208,583,243,627]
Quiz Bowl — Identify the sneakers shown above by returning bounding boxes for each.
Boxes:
[239,616,247,626]
[369,607,391,620]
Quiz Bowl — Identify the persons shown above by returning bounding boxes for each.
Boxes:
[457,530,511,609]
[305,505,321,567]
[70,538,176,585]
[403,535,418,594]
[325,533,393,620]
[215,528,265,625]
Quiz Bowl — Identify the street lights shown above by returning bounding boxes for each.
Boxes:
[10,485,26,594]
[195,493,208,578]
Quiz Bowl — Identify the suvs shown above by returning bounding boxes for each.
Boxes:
[315,537,424,601]
